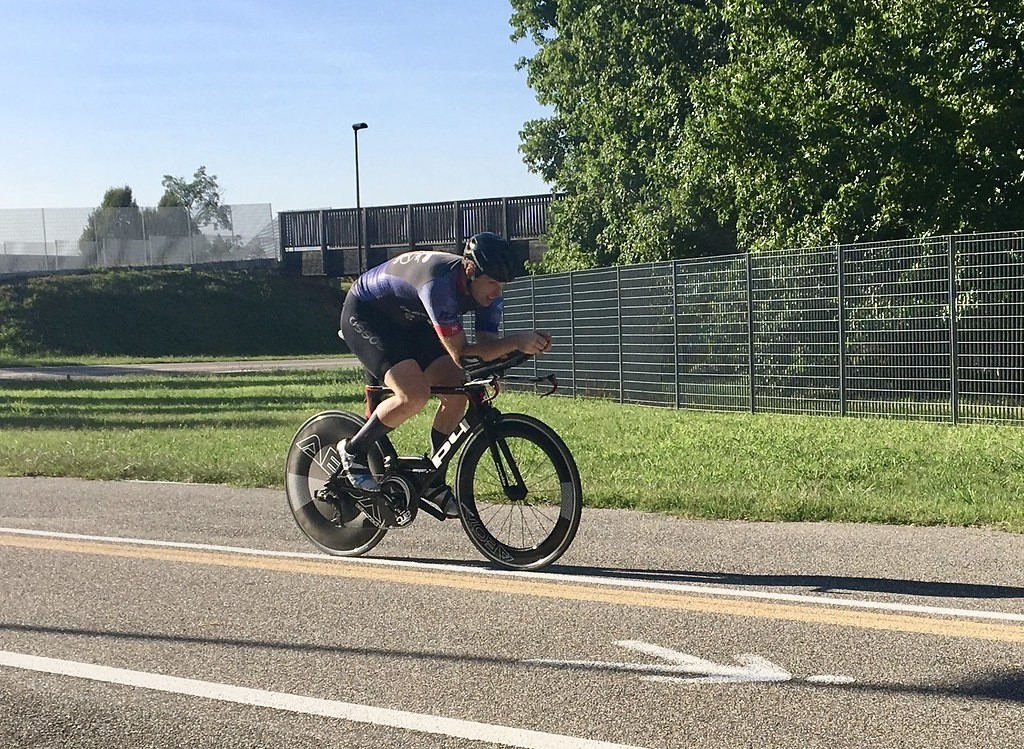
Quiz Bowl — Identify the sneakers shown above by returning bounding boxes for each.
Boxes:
[423,485,458,516]
[336,438,383,493]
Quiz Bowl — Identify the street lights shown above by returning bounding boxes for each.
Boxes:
[352,122,369,279]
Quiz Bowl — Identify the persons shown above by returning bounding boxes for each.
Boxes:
[340,232,551,515]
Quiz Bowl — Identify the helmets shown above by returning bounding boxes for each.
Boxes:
[463,233,517,282]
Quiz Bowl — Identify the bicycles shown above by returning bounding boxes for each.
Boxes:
[284,350,586,573]
